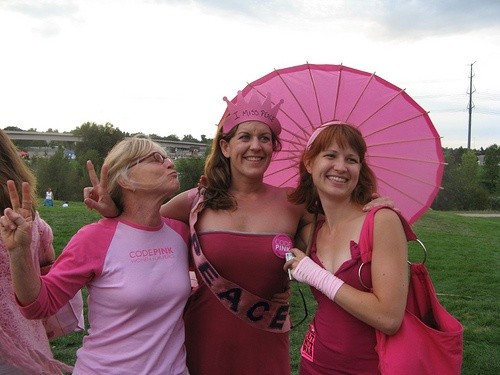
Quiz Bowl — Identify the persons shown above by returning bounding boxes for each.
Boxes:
[61,201,69,208]
[0,132,193,375]
[85,88,401,375]
[283,120,409,375]
[43,187,55,207]
[0,127,74,375]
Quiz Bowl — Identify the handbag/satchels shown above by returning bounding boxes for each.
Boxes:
[358,204,464,375]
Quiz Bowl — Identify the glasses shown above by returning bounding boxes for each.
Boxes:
[128,152,167,170]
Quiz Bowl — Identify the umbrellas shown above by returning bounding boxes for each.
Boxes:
[215,62,448,258]
